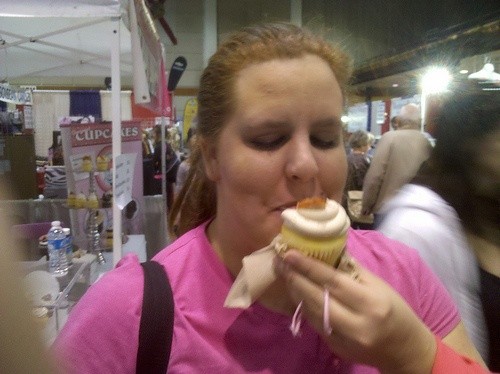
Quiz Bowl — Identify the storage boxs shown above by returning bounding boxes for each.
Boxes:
[0,134,38,200]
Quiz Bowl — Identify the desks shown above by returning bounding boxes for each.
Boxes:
[18,233,146,348]
[0,194,171,259]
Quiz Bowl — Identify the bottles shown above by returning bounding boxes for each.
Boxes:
[47,220,69,277]
[63,228,74,270]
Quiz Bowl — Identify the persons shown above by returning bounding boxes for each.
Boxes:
[391,115,399,130]
[142,124,170,175]
[360,103,436,230]
[374,91,500,374]
[341,129,374,214]
[47,21,492,374]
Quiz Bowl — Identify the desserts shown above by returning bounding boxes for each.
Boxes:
[277,196,351,269]
[67,191,98,209]
[81,155,109,171]
